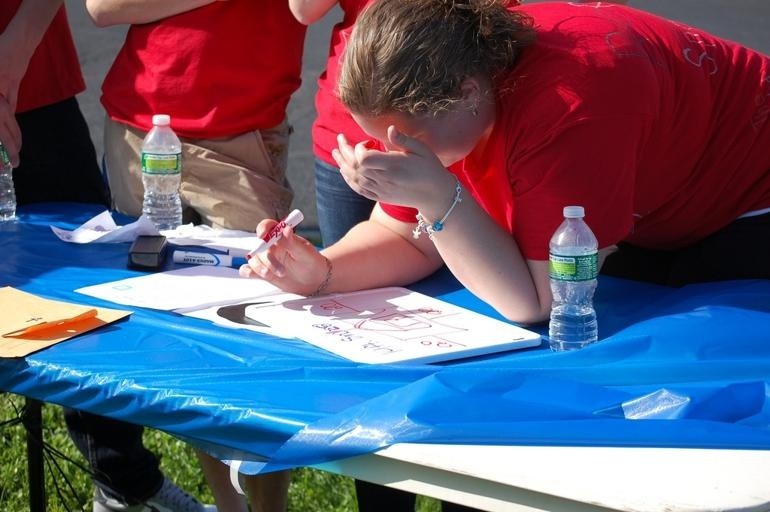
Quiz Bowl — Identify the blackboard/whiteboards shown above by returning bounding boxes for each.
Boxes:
[244,286,543,370]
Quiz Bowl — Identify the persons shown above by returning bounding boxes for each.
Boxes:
[0,1,223,512]
[287,1,483,510]
[237,2,770,326]
[84,1,310,512]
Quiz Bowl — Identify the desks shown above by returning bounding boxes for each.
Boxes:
[1,196,770,511]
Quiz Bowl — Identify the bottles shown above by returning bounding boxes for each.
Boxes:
[0,144,18,222]
[140,114,183,233]
[548,205,599,353]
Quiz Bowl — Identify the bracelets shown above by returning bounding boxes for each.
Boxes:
[410,173,463,240]
[303,243,335,299]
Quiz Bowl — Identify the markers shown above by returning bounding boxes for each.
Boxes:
[245,208,305,261]
[172,249,249,268]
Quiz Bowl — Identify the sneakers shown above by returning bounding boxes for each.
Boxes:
[93,477,218,512]
